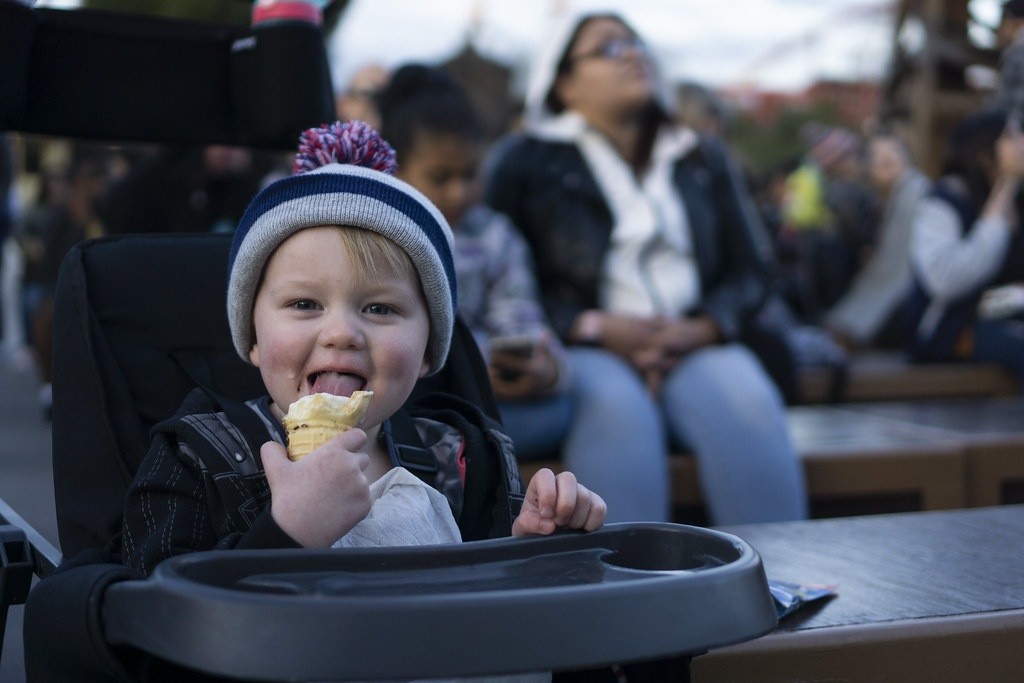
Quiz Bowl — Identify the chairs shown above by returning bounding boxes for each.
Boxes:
[22,234,781,682]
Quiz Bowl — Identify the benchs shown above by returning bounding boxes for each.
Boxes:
[504,334,1024,682]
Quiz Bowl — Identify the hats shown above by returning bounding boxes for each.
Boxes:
[802,121,864,168]
[226,120,458,378]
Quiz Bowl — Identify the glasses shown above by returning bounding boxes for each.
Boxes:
[572,35,645,61]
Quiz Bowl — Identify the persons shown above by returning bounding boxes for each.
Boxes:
[378,0,1023,525]
[120,118,609,580]
[11,140,287,426]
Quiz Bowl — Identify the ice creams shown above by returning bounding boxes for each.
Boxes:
[282,390,374,463]
